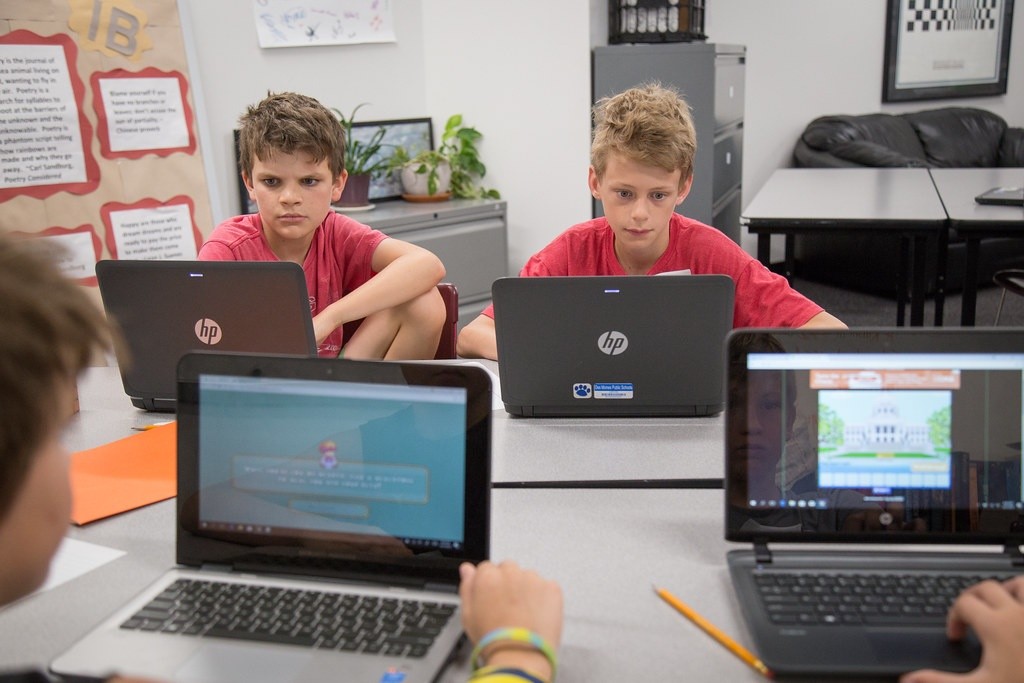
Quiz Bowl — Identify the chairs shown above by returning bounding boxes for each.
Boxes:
[343,282,460,360]
[992,270,1024,327]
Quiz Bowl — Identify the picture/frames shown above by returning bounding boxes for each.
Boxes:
[881,0,1015,104]
[232,117,434,215]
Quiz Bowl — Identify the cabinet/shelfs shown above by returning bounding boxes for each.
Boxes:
[346,200,509,336]
[593,43,747,247]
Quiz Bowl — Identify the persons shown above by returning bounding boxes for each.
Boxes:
[457,84,848,361]
[902,576,1024,683]
[0,231,565,683]
[198,93,446,362]
[729,332,882,510]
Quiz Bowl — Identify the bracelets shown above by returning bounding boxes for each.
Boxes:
[464,627,558,683]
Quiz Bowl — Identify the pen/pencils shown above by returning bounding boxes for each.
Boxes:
[652,583,774,679]
[131,425,159,431]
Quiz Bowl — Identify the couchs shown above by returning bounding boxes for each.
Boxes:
[791,107,1024,303]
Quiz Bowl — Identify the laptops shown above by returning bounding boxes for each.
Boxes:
[48,350,493,683]
[975,186,1024,206]
[722,326,1024,683]
[492,275,736,419]
[95,258,317,414]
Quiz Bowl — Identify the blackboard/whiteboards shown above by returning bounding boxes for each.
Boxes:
[252,0,396,48]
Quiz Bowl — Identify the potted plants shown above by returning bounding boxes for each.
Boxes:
[390,113,500,203]
[329,101,394,215]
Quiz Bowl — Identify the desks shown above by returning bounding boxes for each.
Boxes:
[0,485,1003,683]
[929,166,1024,327]
[739,166,948,327]
[61,365,725,488]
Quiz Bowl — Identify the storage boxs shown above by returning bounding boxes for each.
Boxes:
[607,0,709,45]
